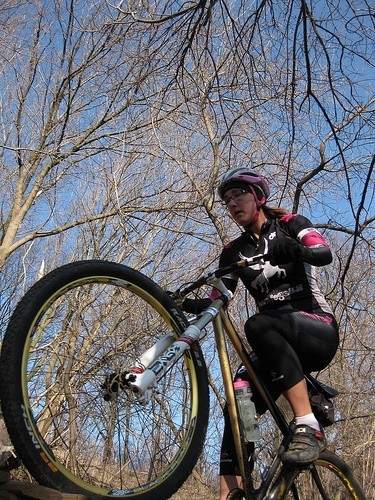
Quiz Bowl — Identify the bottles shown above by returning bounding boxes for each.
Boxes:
[234,378,260,442]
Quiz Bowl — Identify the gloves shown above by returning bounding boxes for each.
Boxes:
[269,237,303,266]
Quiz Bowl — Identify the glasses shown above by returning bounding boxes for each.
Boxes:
[218,188,252,203]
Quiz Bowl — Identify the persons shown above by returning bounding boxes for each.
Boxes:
[166,167,340,500]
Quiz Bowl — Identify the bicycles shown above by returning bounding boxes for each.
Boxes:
[0,255,367,500]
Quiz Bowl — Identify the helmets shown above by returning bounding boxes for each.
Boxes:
[217,166,271,200]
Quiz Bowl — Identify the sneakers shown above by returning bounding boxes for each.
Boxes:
[284,422,330,461]
